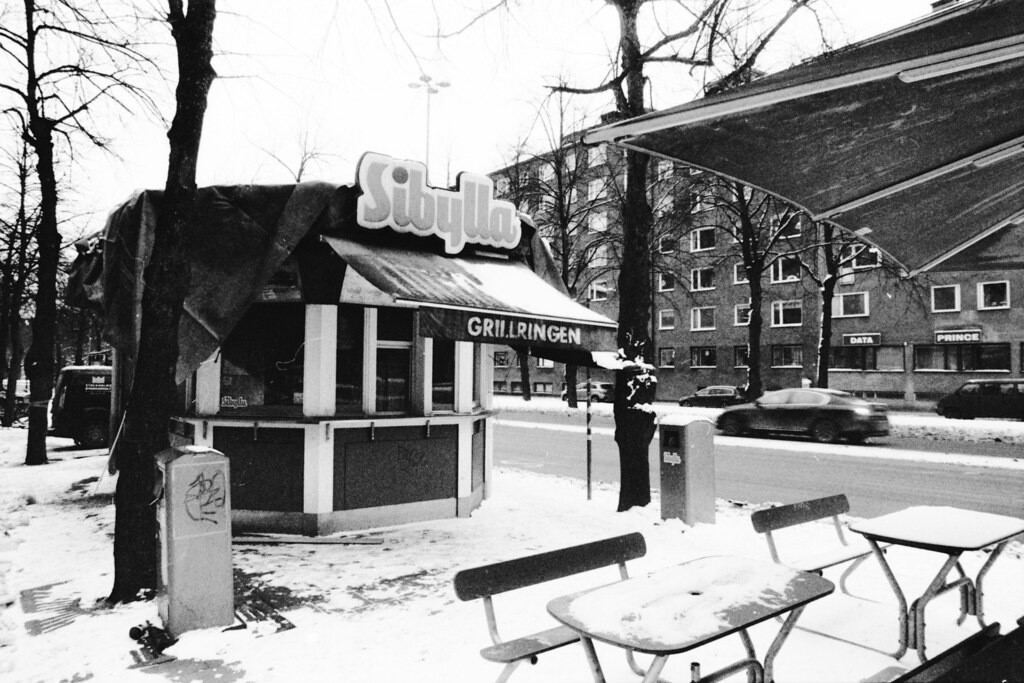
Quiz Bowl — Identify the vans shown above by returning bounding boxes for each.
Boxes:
[935,378,1024,420]
[46,366,112,446]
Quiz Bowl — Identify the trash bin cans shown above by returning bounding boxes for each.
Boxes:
[153,445,234,639]
[658,412,719,525]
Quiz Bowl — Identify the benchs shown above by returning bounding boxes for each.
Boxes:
[750,493,894,655]
[453,531,647,683]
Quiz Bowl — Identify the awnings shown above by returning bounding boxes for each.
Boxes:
[578,0,1024,276]
[322,237,618,354]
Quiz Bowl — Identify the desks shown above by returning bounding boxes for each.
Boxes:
[547,553,835,683]
[847,505,1024,665]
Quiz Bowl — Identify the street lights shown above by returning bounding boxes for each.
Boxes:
[408,72,451,165]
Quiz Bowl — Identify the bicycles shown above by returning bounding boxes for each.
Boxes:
[0,396,30,424]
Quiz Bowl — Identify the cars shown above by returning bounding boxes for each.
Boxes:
[715,386,891,444]
[560,382,615,402]
[679,384,745,407]
